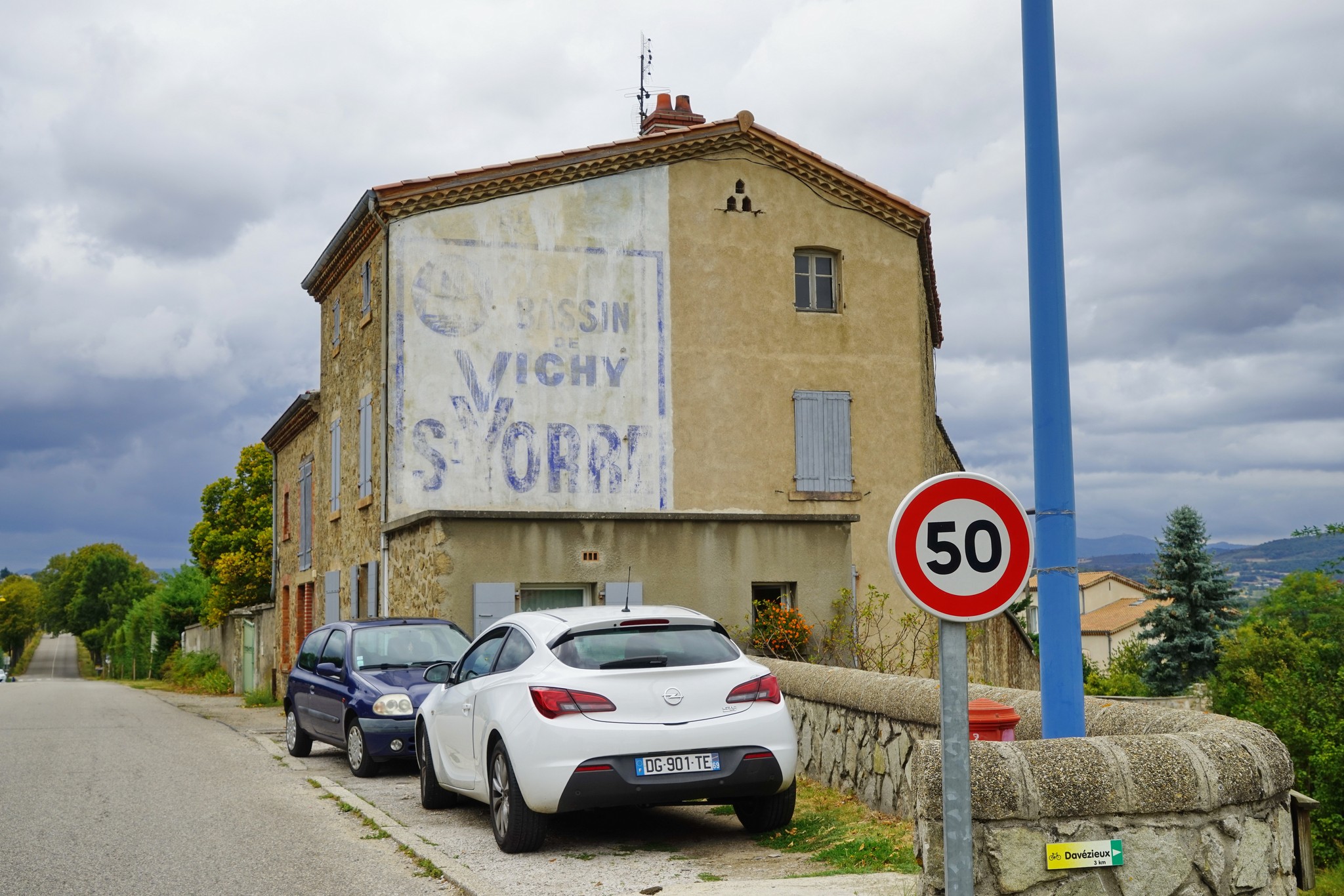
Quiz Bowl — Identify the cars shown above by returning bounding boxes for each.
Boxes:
[0,669,8,683]
[414,567,798,857]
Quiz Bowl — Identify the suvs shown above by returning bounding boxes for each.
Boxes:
[282,589,505,777]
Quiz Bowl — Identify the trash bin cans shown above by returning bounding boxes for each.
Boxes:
[968,697,1019,741]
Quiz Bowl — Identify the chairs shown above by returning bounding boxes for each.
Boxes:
[389,637,435,663]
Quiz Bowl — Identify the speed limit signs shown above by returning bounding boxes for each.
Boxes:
[887,472,1034,622]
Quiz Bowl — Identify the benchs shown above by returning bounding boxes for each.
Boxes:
[560,633,721,669]
